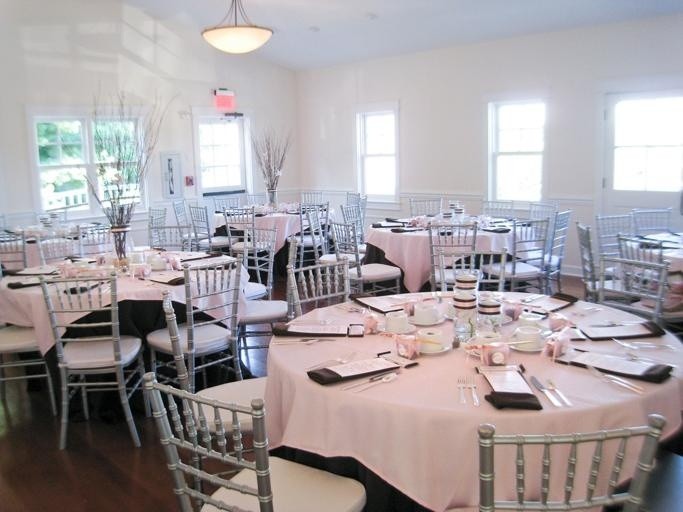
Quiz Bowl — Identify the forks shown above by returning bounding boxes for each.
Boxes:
[546,375,568,407]
[456,372,468,406]
[611,338,676,351]
[466,374,480,406]
[271,339,317,347]
[304,353,355,372]
[587,364,643,395]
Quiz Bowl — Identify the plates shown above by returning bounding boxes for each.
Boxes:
[502,315,513,325]
[417,340,450,357]
[441,306,453,321]
[382,324,415,335]
[508,342,544,353]
[464,345,483,356]
[408,314,446,327]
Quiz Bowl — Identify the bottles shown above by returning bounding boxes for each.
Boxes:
[452,274,501,341]
[38,210,58,233]
[442,199,464,223]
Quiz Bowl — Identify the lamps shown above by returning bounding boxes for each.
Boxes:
[199,1,275,55]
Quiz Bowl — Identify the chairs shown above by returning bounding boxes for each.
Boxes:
[147,254,244,405]
[443,414,667,511]
[142,370,368,511]
[162,289,271,508]
[1,322,68,435]
[2,189,681,329]
[38,272,152,448]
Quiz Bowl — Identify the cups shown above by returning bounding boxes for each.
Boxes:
[169,256,180,270]
[517,313,542,327]
[363,313,378,335]
[467,332,501,353]
[487,342,507,366]
[415,216,423,228]
[95,250,166,280]
[413,303,440,320]
[384,312,408,332]
[395,334,416,360]
[417,328,444,350]
[510,327,541,348]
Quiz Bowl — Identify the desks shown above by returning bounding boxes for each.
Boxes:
[261,290,680,510]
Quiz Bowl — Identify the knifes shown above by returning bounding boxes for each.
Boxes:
[272,338,335,343]
[334,303,360,313]
[520,293,545,303]
[338,370,400,394]
[529,375,562,410]
